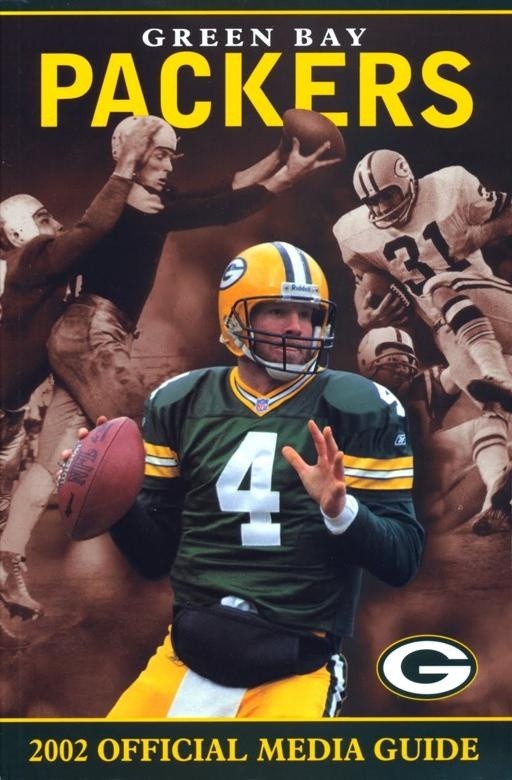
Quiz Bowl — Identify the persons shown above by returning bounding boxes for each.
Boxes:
[2,109,511,722]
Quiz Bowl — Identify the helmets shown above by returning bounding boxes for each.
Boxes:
[0,194,44,249]
[353,149,417,231]
[111,116,178,167]
[358,325,419,391]
[216,238,338,383]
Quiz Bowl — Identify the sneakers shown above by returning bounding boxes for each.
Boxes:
[466,377,512,414]
[0,549,45,622]
[472,471,512,537]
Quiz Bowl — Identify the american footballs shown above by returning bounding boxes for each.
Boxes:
[57,417,143,539]
[281,110,344,160]
[364,267,415,328]
